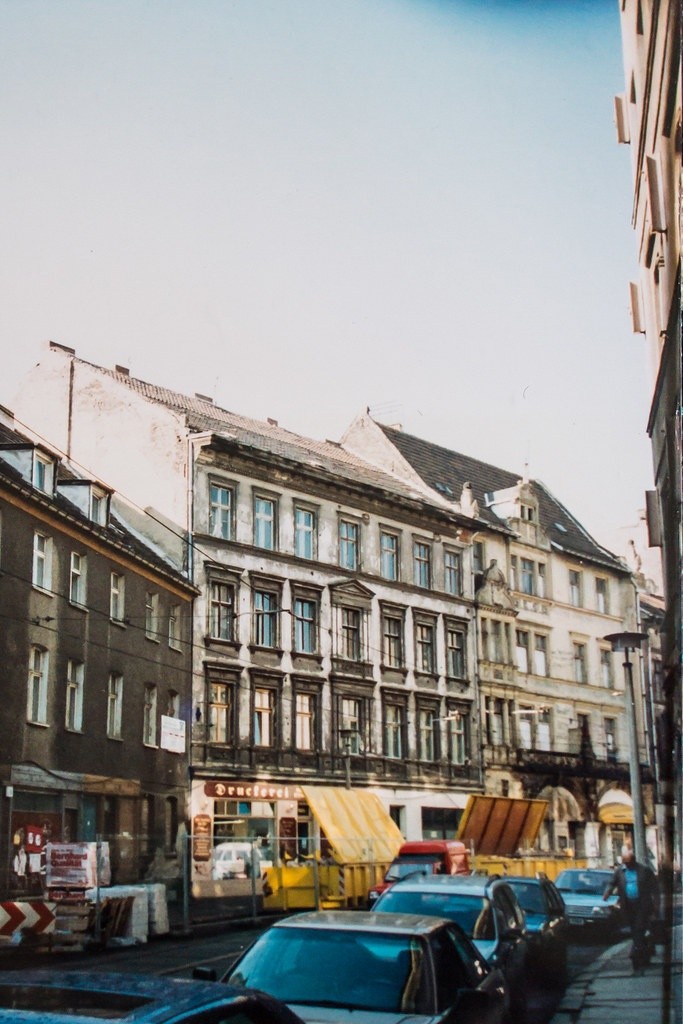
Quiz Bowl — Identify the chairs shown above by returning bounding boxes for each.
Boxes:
[286,974,331,1000]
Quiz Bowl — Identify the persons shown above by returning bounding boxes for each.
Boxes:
[602,852,661,978]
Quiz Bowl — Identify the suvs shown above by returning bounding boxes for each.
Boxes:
[369,870,530,992]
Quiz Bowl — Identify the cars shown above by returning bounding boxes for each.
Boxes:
[190,909,513,1024]
[0,967,306,1024]
[504,872,571,966]
[555,868,625,941]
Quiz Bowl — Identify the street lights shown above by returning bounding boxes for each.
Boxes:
[602,630,652,865]
[335,725,359,789]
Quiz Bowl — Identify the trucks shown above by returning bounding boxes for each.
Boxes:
[365,838,489,910]
